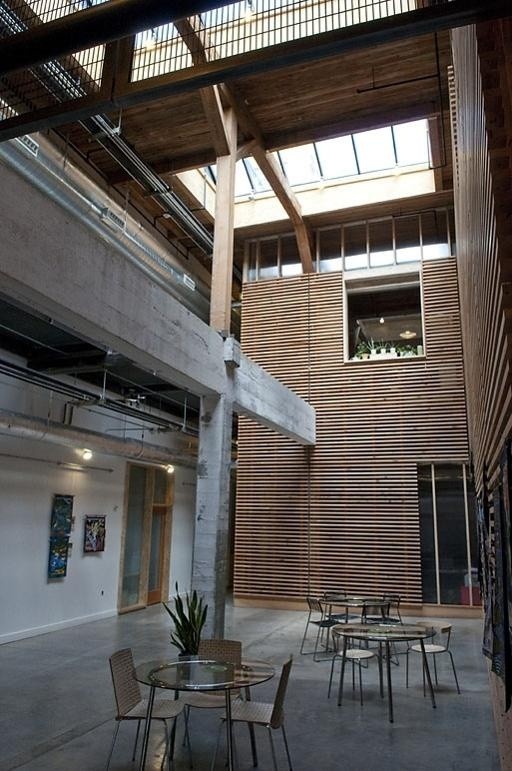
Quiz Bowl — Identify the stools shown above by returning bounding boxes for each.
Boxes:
[328,627,380,706]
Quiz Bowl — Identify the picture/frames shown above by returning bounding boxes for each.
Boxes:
[48,494,73,580]
[83,517,105,552]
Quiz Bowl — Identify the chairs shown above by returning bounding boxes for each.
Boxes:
[183,640,242,746]
[211,653,293,771]
[322,591,359,650]
[406,621,461,697]
[359,593,409,649]
[106,648,185,770]
[300,598,342,662]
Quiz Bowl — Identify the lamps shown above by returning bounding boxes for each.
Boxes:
[399,329,416,339]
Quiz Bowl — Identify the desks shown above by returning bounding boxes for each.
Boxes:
[320,599,391,650]
[133,656,275,771]
[334,624,437,722]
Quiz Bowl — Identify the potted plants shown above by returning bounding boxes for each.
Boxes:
[350,336,417,360]
[161,582,207,677]
